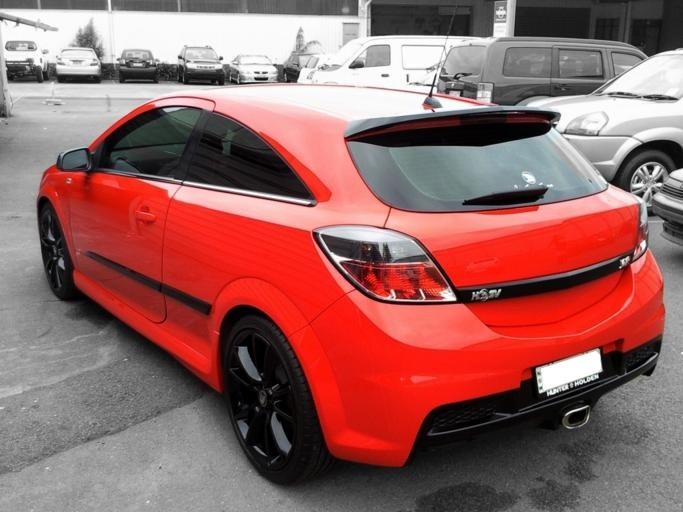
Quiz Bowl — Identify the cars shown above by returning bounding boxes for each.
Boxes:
[33,80,668,493]
